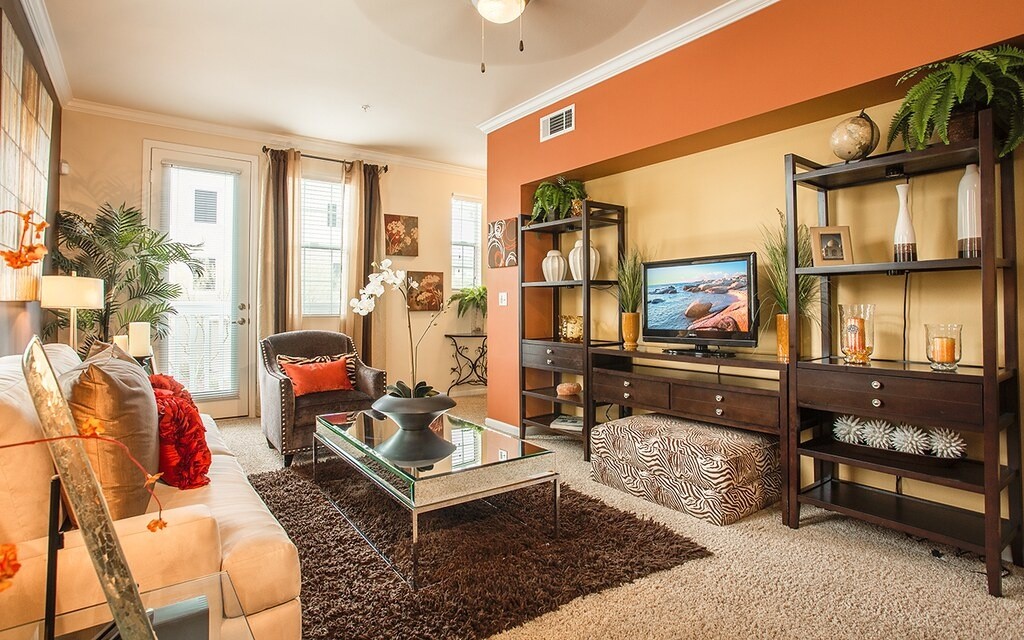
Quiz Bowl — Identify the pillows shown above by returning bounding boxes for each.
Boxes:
[273,350,360,395]
[146,368,215,494]
[285,359,354,399]
[56,337,162,526]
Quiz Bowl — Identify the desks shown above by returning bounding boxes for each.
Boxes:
[443,332,487,397]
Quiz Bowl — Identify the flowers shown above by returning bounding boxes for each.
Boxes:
[349,254,457,398]
[0,413,170,535]
[0,203,53,271]
[0,535,26,587]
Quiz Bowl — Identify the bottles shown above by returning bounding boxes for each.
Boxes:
[542,250,568,281]
[569,239,601,281]
[958,164,980,259]
[893,184,917,262]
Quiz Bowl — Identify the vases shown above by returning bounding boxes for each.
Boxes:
[892,181,919,263]
[953,159,982,262]
[370,389,458,433]
[365,430,460,471]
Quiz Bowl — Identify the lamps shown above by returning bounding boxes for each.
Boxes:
[37,268,106,351]
[465,0,536,78]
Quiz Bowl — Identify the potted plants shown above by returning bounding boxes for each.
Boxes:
[744,205,836,364]
[876,41,1024,155]
[445,276,489,334]
[595,240,658,351]
[525,176,589,230]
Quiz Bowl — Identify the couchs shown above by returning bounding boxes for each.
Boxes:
[254,327,392,469]
[0,339,306,640]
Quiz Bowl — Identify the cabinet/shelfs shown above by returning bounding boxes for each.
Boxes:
[512,198,630,469]
[787,107,1024,599]
[585,336,788,529]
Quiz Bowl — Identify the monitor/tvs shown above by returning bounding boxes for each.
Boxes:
[640,250,760,358]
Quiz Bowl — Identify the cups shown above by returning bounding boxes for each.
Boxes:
[838,304,876,364]
[924,323,963,372]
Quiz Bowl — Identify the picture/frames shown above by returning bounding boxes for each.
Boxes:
[808,223,856,267]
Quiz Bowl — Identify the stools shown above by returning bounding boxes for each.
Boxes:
[589,411,780,529]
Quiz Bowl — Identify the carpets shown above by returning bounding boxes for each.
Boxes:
[246,456,1024,640]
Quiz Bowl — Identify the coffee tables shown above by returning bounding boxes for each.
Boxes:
[309,408,565,596]
